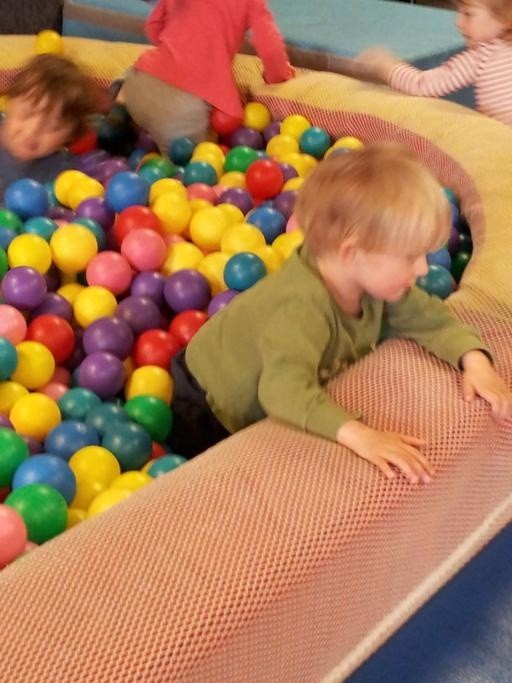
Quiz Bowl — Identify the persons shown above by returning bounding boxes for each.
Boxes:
[1,54,92,190]
[93,2,294,164]
[162,138,512,492]
[356,0,512,133]
